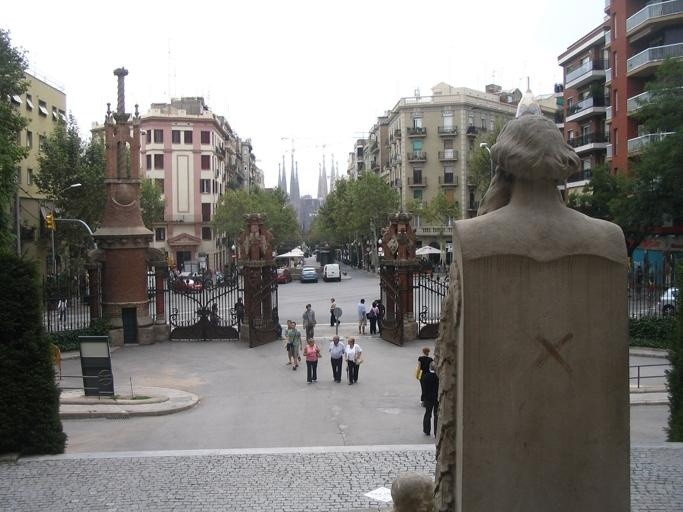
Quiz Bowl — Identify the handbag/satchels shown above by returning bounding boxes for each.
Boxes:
[355,345,364,365]
[308,321,315,328]
[316,346,322,357]
[287,342,294,351]
[367,310,374,319]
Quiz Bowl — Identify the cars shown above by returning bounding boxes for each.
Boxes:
[274,268,291,284]
[657,286,678,316]
[299,266,318,283]
[166,268,225,294]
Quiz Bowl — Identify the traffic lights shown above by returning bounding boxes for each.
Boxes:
[45,215,53,229]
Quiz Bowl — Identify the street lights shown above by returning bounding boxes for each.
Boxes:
[50,182,81,273]
[480,142,494,179]
[230,244,236,287]
[344,239,382,272]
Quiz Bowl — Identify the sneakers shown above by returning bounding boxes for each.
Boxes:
[330,321,341,326]
[298,356,301,361]
[419,401,424,407]
[348,382,353,385]
[286,362,291,365]
[312,379,317,382]
[354,380,356,383]
[292,364,299,370]
[306,381,311,384]
[424,431,436,439]
[357,330,381,335]
[334,379,341,383]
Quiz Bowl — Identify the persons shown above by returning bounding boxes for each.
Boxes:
[283,297,385,386]
[626,265,655,307]
[235,297,245,333]
[415,346,433,407]
[420,361,440,439]
[56,294,71,324]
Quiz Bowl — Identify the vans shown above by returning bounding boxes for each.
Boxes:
[322,262,341,281]
